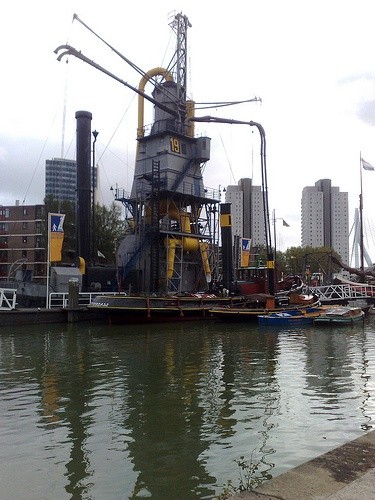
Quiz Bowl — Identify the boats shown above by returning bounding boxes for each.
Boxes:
[86,152,375,326]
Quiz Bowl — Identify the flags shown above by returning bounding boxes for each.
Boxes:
[362,158,374,171]
